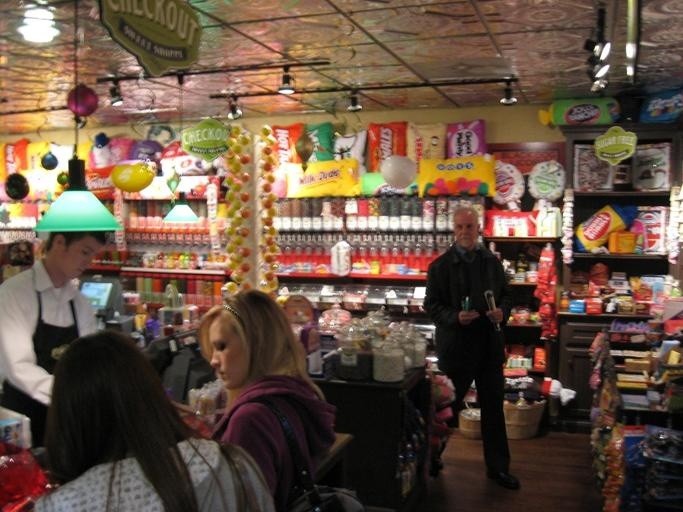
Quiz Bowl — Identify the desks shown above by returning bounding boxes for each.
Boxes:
[160,404,353,484]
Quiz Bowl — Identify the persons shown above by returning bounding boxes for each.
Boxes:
[199,291,336,512]
[35,330,276,512]
[0,231,109,448]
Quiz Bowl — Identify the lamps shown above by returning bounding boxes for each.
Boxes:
[225,98,241,124]
[108,87,126,109]
[581,37,612,62]
[344,94,362,116]
[586,58,610,78]
[497,77,517,109]
[31,159,123,232]
[276,74,296,96]
[162,193,204,228]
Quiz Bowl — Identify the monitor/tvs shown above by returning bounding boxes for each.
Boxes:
[142,326,219,405]
[77,277,124,316]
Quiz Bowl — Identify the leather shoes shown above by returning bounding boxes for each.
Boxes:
[484,465,520,490]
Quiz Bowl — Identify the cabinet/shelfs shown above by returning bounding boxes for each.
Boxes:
[267,143,564,403]
[554,187,678,428]
[0,199,236,334]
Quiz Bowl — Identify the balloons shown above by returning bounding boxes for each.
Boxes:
[5,174,30,198]
[259,124,279,290]
[41,152,59,171]
[112,160,155,192]
[219,123,252,292]
[380,156,416,189]
[295,134,313,160]
[68,85,98,118]
[424,208,521,491]
